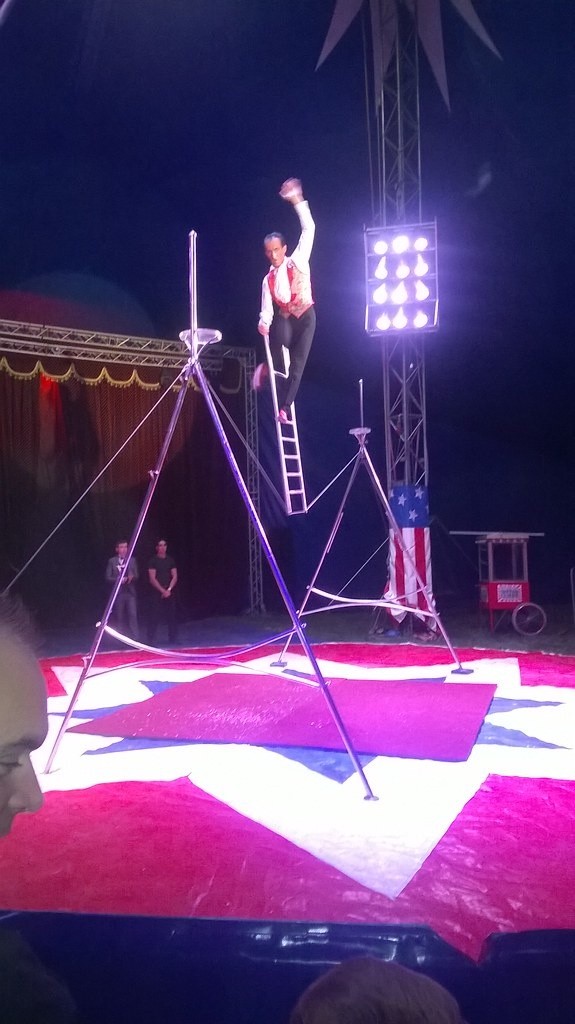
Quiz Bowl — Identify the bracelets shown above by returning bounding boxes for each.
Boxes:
[164,587,173,592]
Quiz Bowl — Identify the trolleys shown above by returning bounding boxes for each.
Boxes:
[472,533,547,636]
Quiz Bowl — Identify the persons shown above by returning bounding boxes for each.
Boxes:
[148,537,188,645]
[0,592,87,1024]
[246,179,317,423]
[103,540,144,647]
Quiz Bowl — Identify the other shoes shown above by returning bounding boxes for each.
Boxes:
[278,406,289,426]
[253,363,266,390]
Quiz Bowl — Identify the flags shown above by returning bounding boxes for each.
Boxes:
[382,483,444,640]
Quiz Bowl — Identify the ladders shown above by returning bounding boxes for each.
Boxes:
[262,335,308,516]
